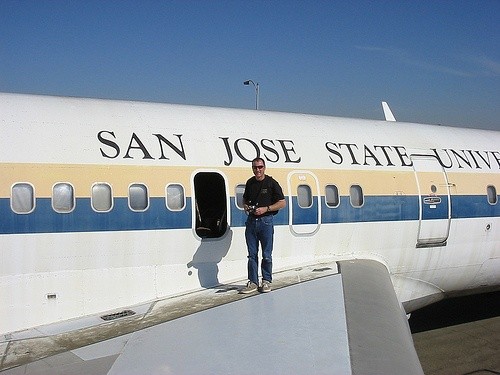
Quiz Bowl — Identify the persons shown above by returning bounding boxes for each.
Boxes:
[239,158,286,294]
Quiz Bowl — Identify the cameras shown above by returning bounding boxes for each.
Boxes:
[248,206,257,215]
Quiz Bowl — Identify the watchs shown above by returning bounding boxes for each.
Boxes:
[267,206,271,212]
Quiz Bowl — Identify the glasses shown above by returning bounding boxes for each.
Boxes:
[252,166,264,169]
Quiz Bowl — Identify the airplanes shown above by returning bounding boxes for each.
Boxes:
[0,92,500,375]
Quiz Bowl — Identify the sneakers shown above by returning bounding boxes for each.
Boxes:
[262,280,272,292]
[240,281,259,293]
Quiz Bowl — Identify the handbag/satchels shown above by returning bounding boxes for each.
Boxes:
[268,177,279,215]
